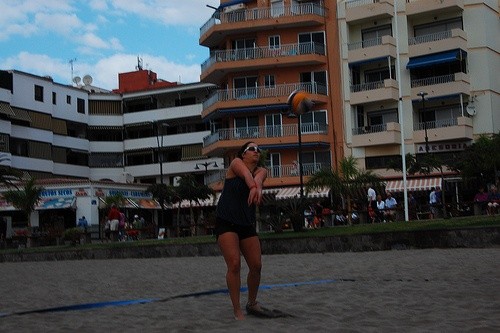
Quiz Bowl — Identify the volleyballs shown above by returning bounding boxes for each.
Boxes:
[286,90,313,117]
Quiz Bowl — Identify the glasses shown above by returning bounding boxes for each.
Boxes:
[244,146,261,153]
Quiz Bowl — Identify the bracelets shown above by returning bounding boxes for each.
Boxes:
[249,185,257,189]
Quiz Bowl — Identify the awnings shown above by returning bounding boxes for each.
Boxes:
[0,196,162,211]
[164,176,443,209]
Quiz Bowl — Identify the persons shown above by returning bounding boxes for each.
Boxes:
[304,183,500,222]
[215,141,268,321]
[78,216,89,232]
[104,203,145,241]
